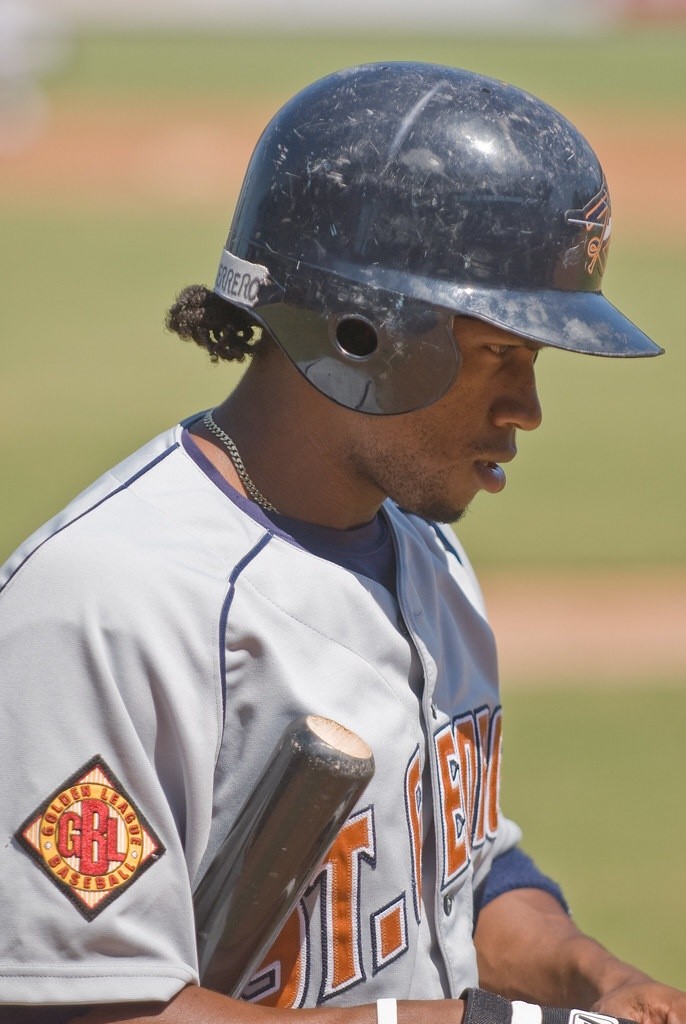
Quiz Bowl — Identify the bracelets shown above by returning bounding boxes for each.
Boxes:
[376,998,397,1024]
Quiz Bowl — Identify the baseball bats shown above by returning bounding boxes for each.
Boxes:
[191,715,376,999]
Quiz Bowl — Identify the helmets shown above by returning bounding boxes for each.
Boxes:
[213,60,667,417]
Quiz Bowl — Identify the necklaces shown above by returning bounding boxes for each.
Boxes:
[203,406,281,514]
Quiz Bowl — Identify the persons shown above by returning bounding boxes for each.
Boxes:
[0,63,686,1024]
